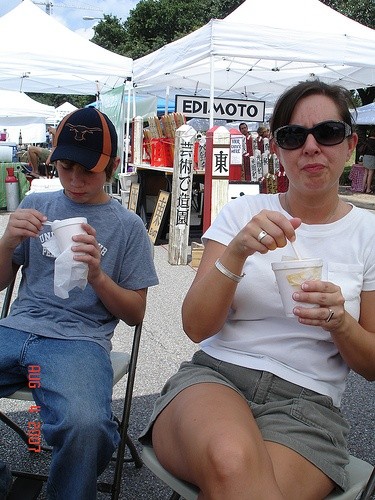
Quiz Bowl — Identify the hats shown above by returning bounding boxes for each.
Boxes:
[45,106,118,173]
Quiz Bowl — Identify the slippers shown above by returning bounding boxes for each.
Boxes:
[25,172,39,179]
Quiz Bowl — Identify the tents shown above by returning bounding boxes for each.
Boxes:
[0,0,375,121]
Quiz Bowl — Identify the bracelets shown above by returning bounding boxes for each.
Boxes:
[214,257,246,283]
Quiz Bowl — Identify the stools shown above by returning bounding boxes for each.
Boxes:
[42,161,59,179]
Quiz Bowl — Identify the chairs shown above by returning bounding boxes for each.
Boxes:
[134,381,375,500]
[0,235,155,500]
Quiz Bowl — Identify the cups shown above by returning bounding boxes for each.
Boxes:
[271,257,324,320]
[51,217,89,267]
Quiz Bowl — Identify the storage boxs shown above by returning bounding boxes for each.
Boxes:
[118,172,139,191]
[0,143,16,163]
[119,188,131,209]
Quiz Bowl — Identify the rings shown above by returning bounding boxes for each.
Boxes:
[256,230,267,241]
[326,307,334,323]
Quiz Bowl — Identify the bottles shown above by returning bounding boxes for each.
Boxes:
[19,132,22,145]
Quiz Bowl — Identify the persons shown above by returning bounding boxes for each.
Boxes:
[238,122,275,161]
[0,105,161,500]
[24,126,57,179]
[360,130,375,195]
[138,78,375,500]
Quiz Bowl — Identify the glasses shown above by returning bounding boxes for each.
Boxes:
[274,119,352,150]
